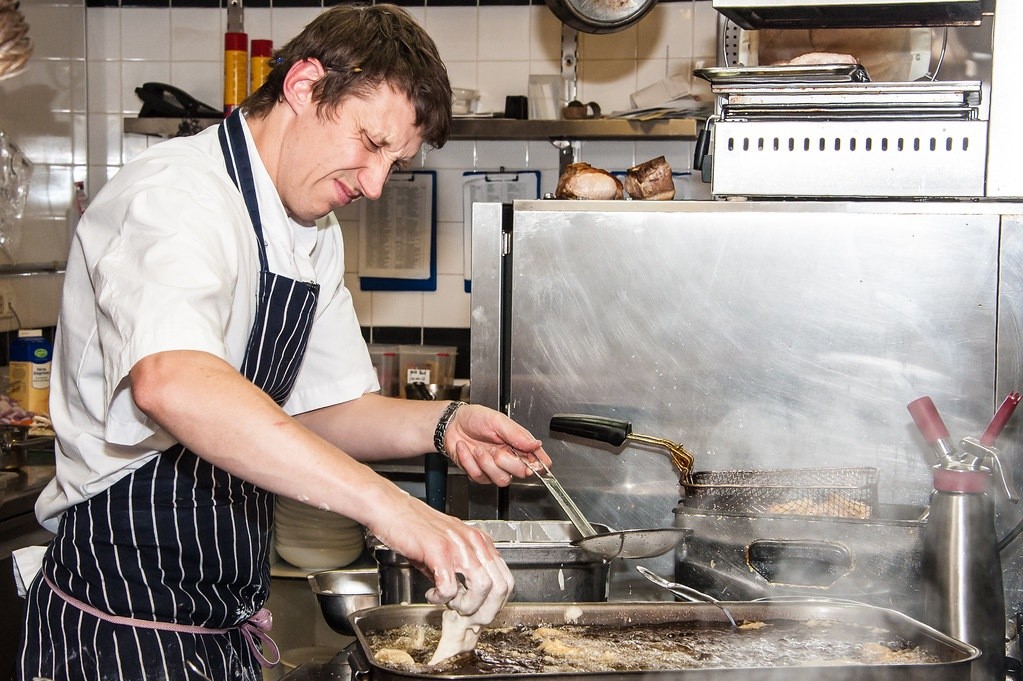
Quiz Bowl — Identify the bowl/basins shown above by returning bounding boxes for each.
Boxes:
[373,519,614,606]
[275,496,366,569]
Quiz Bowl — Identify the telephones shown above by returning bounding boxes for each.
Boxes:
[133,82,226,118]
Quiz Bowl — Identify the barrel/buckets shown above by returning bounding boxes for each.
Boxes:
[279,645,344,674]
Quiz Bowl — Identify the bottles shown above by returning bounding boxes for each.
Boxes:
[915,464,1005,681]
[8,328,51,414]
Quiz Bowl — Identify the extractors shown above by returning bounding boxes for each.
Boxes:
[713,0,996,29]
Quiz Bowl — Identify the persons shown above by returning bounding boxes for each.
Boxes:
[11,3,553,681]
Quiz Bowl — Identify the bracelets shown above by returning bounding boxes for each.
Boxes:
[434,401,468,458]
